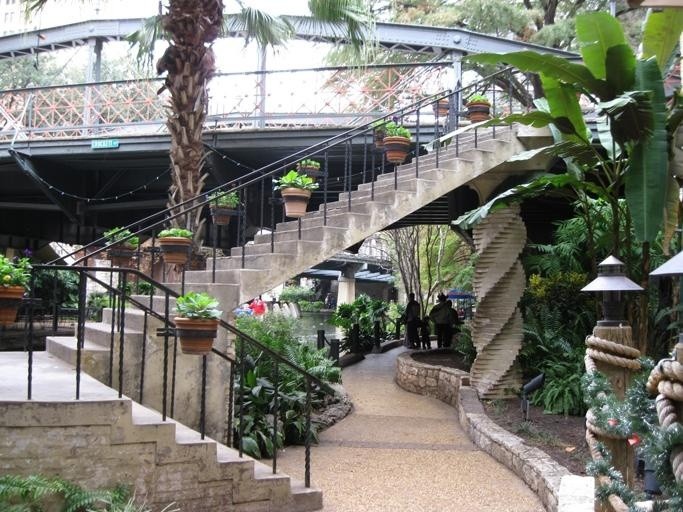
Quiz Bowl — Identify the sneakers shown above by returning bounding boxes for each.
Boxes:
[405,344,431,350]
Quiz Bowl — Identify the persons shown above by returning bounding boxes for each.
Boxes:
[330,296,336,309]
[399,309,408,346]
[457,307,465,316]
[443,300,458,348]
[406,293,421,349]
[248,296,265,323]
[325,293,332,309]
[418,317,432,350]
[429,295,451,348]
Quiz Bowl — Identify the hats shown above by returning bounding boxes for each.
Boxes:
[437,293,449,301]
[422,315,431,321]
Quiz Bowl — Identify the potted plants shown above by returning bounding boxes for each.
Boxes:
[383,124,410,164]
[272,170,319,220]
[295,159,319,179]
[158,227,194,265]
[430,95,449,117]
[466,94,490,122]
[103,227,139,266]
[207,191,238,227]
[173,289,220,354]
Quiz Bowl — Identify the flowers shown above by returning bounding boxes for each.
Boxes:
[371,114,398,131]
[0,249,34,286]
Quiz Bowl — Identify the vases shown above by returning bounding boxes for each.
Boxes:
[0,284,26,325]
[373,131,385,149]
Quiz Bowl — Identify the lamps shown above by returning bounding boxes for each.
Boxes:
[577,254,646,325]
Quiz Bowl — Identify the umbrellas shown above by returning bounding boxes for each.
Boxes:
[445,289,473,298]
